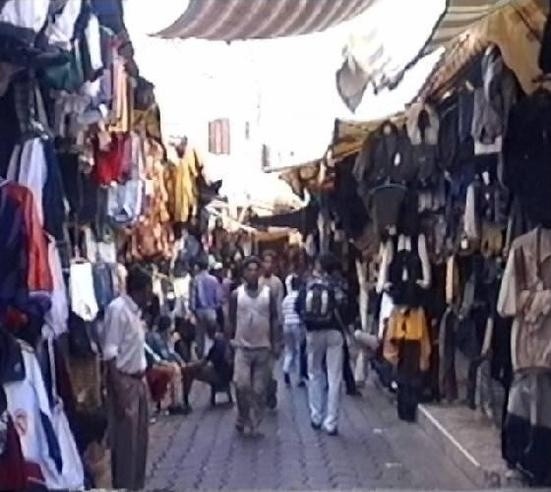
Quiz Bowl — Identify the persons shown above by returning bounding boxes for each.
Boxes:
[280,276,306,387]
[224,257,281,439]
[181,326,234,411]
[141,318,191,415]
[188,257,223,359]
[294,254,356,435]
[102,263,153,492]
[258,248,285,410]
[146,315,180,362]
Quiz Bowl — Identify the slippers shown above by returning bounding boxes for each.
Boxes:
[299,277,338,331]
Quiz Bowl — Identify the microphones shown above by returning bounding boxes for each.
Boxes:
[210,384,233,407]
